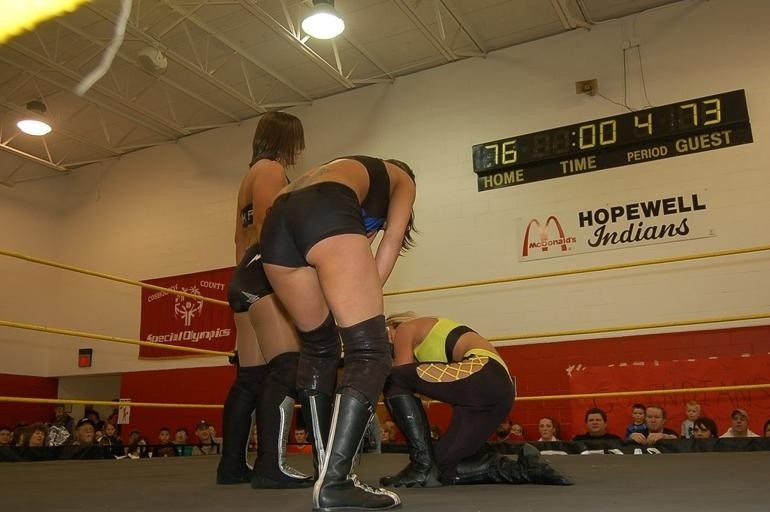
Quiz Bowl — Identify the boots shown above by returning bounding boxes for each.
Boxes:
[250,381,316,490]
[216,379,256,486]
[312,393,403,512]
[299,385,332,482]
[436,441,572,486]
[378,393,440,490]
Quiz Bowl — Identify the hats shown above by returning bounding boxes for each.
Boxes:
[76,418,97,427]
[731,408,747,418]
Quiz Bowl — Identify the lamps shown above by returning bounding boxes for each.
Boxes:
[15,100,55,138]
[295,0,347,41]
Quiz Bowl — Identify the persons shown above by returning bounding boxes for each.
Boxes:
[216,111,317,488]
[380,310,575,489]
[260,155,421,512]
